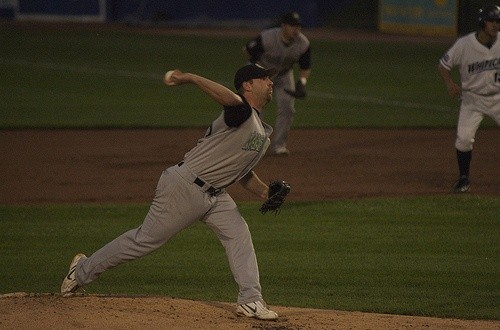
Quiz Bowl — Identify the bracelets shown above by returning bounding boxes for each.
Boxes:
[300,77,307,85]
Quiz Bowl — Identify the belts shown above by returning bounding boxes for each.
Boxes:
[178,162,221,197]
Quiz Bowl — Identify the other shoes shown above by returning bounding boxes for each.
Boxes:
[453,177,470,191]
[273,143,290,154]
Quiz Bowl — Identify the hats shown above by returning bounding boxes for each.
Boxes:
[280,11,305,27]
[234,64,278,90]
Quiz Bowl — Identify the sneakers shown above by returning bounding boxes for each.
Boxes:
[61,253,88,298]
[236,299,279,320]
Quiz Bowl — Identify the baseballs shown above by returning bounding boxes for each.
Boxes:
[164,71,174,85]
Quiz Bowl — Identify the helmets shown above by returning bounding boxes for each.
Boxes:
[478,4,500,28]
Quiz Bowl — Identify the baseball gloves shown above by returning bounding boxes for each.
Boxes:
[259,181,292,215]
[284,79,306,100]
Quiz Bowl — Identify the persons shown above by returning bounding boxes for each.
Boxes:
[60,63,290,320]
[242,11,311,155]
[437,4,500,192]
